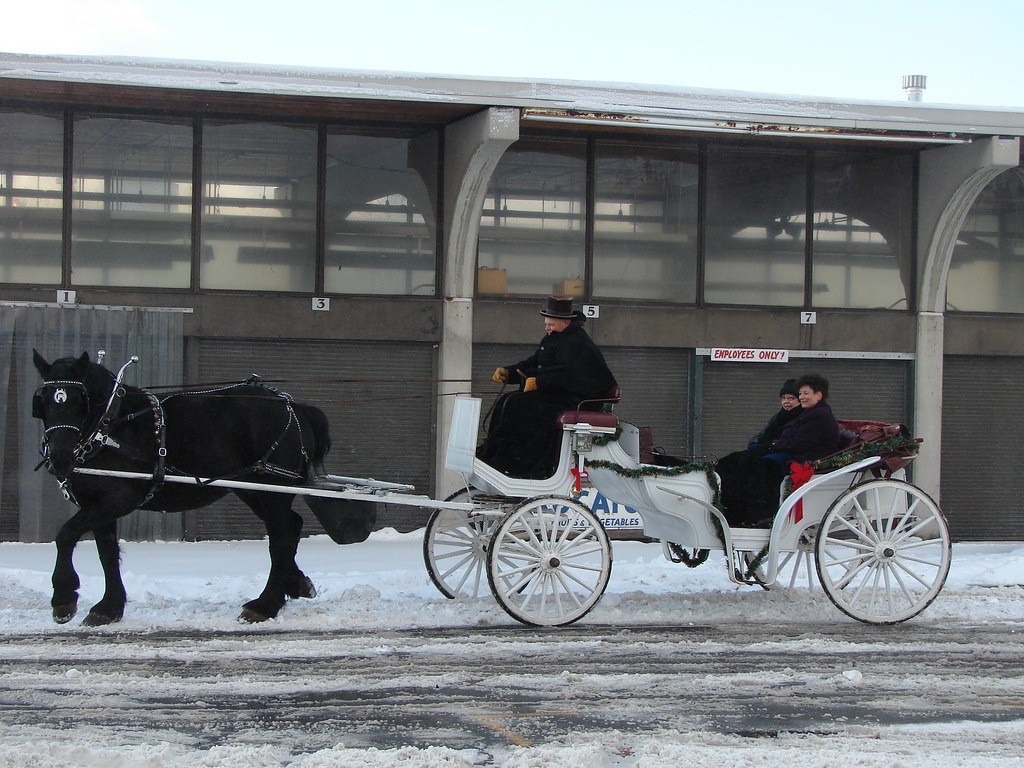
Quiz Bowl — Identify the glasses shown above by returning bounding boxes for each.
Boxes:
[781,397,797,401]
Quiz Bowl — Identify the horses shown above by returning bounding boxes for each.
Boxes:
[31,347,332,627]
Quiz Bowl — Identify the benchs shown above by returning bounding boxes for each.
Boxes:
[557,385,623,434]
[813,431,857,475]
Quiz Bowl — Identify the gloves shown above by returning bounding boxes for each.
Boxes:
[491,368,509,385]
[524,377,537,392]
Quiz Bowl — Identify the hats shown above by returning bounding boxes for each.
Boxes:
[780,379,799,398]
[539,296,578,319]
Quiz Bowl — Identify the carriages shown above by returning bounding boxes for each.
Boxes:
[27,343,954,629]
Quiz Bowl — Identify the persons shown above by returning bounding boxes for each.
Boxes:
[715,373,840,526]
[481,294,604,476]
